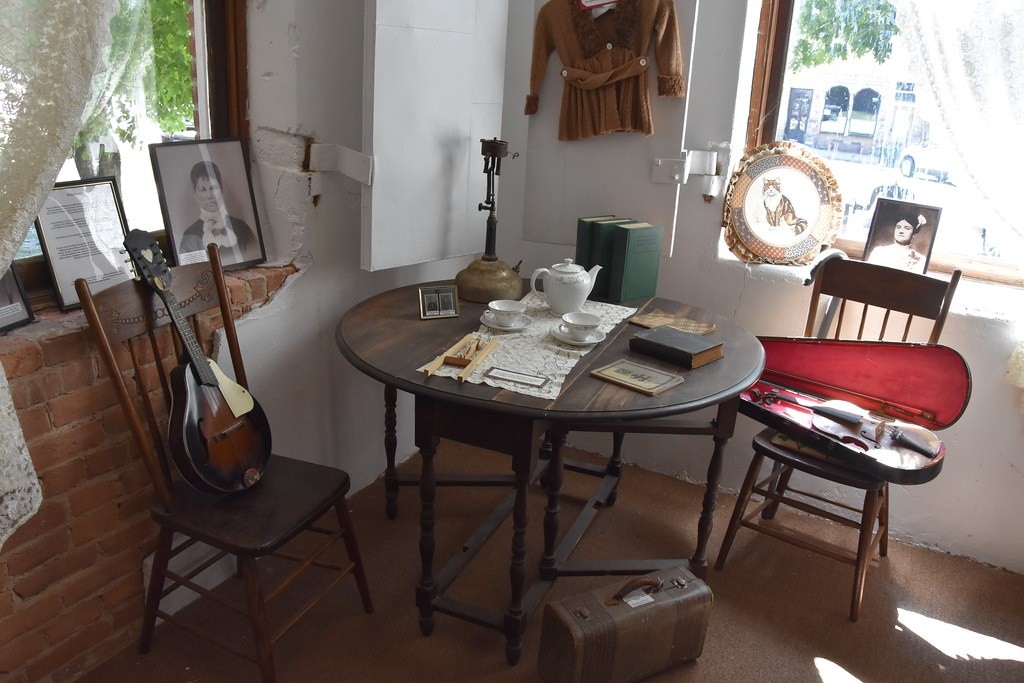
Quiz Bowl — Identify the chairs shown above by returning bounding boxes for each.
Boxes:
[73,242,374,683]
[712,243,962,624]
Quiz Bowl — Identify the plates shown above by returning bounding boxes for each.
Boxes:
[480,312,533,331]
[549,324,606,346]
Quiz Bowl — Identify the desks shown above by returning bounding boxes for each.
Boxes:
[332,273,766,666]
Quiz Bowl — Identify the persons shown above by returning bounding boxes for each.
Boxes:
[868,215,926,275]
[442,295,452,309]
[427,296,436,310]
[180,161,258,266]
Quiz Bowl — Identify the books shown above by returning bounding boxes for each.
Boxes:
[576,214,664,303]
[591,359,684,397]
[628,308,716,336]
[630,325,725,370]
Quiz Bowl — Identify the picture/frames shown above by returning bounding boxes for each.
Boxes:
[418,284,459,320]
[0,261,35,332]
[34,176,141,315]
[862,197,943,274]
[147,134,268,271]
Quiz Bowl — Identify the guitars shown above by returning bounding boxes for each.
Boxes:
[118,229,272,494]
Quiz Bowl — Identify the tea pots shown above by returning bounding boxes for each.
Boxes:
[530,258,603,314]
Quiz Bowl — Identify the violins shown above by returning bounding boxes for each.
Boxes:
[748,386,943,471]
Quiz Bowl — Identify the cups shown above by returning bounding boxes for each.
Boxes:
[559,312,601,342]
[483,299,526,327]
